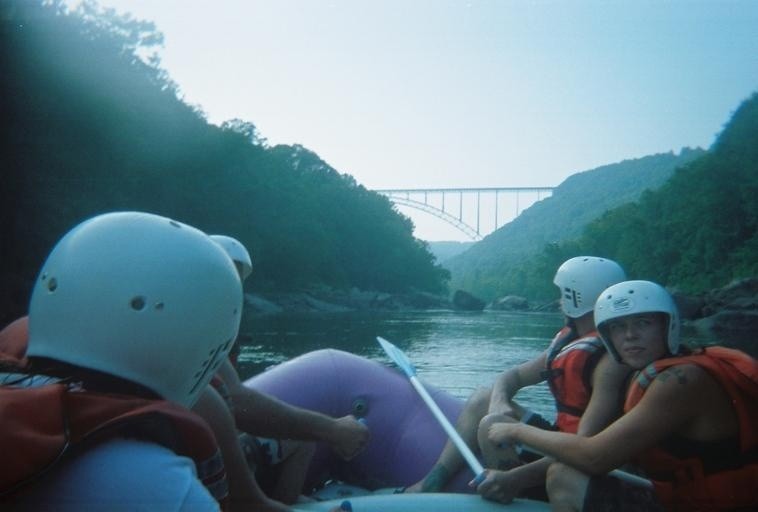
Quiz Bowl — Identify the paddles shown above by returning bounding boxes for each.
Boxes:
[376,336,484,478]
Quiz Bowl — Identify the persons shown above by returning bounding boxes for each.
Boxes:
[192,372,295,512]
[487,279,758,511]
[0,212,244,512]
[206,234,372,507]
[371,256,627,505]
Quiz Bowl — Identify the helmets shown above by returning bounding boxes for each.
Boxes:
[26,210,243,412]
[593,280,680,363]
[207,234,252,281]
[553,256,626,319]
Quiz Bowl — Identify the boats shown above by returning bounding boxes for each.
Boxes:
[221,345,553,511]
